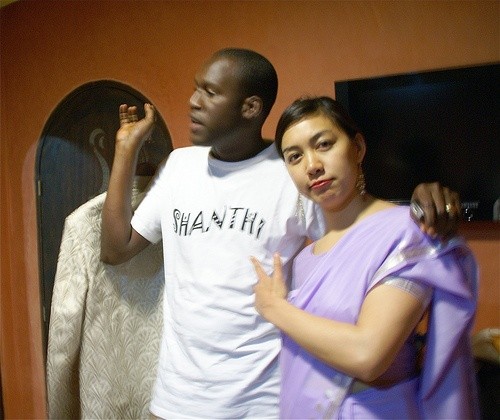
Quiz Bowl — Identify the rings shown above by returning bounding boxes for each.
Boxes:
[447,203,457,214]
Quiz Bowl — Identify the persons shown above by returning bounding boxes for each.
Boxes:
[249,94,483,419]
[98,46,463,419]
[44,120,167,420]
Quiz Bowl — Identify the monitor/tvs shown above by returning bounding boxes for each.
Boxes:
[333,60,500,222]
